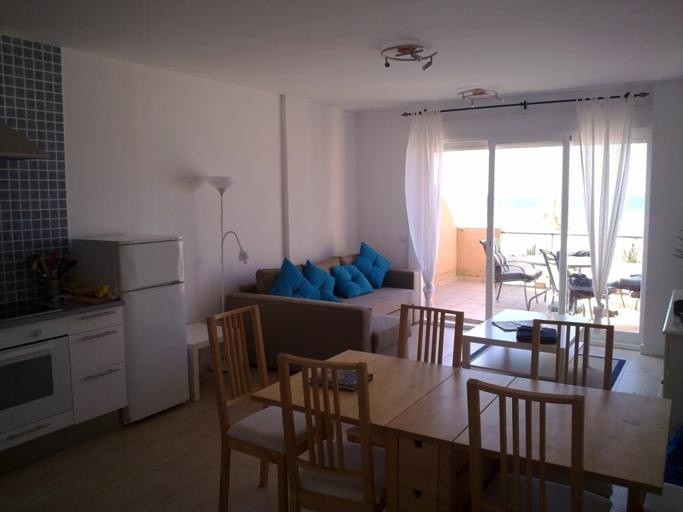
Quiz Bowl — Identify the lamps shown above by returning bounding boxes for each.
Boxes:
[380,44,438,72]
[458,87,504,108]
[206,174,250,313]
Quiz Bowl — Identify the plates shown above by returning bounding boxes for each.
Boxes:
[307,371,373,391]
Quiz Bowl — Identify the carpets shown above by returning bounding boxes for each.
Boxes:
[470,343,626,390]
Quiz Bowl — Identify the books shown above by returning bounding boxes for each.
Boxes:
[309,365,374,391]
[517,328,556,343]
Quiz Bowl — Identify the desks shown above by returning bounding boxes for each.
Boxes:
[567,255,592,275]
[455,377,673,512]
[252,350,460,428]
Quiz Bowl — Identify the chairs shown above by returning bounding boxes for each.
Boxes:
[207,305,328,512]
[613,275,641,310]
[530,318,614,390]
[480,238,542,307]
[539,249,595,320]
[466,379,613,512]
[346,303,464,448]
[277,352,387,511]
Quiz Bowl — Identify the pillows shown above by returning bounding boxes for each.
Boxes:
[354,242,392,288]
[272,258,321,299]
[331,265,374,298]
[302,260,336,299]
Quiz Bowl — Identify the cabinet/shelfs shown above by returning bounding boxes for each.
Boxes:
[662,288,683,434]
[67,306,129,426]
[386,369,514,512]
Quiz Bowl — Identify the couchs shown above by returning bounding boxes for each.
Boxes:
[226,255,424,373]
